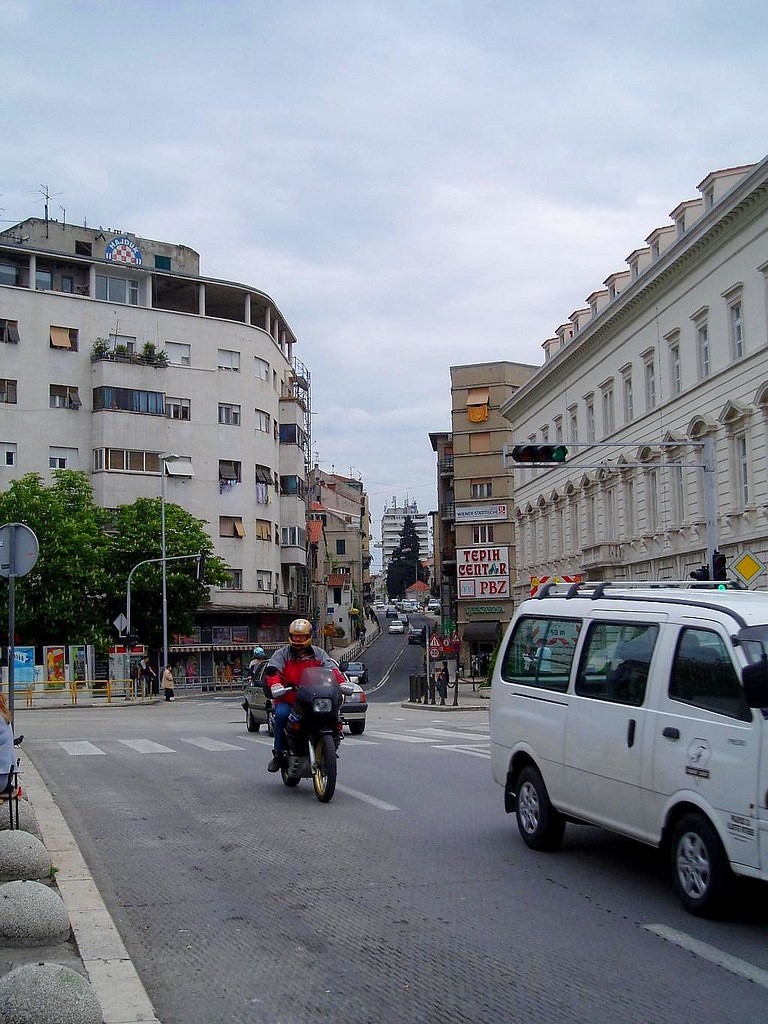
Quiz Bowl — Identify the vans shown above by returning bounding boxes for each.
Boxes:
[488,578,768,917]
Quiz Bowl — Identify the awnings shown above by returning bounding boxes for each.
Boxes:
[463,622,501,641]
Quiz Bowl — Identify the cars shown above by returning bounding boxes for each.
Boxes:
[374,598,441,624]
[408,627,423,644]
[345,661,370,684]
[388,620,404,634]
[241,660,369,738]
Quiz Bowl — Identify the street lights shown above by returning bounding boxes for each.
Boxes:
[162,450,178,669]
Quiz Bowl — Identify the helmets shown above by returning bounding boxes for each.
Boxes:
[253,647,264,655]
[288,618,314,649]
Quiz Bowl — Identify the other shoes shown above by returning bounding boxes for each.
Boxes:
[268,757,280,772]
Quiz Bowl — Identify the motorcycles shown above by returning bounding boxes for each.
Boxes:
[262,659,352,804]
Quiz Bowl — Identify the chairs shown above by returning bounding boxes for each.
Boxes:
[0,758,20,830]
[682,648,735,712]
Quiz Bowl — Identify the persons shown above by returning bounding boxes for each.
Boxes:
[161,665,174,701]
[355,624,366,641]
[137,656,156,696]
[534,638,552,677]
[437,660,449,705]
[249,648,265,673]
[365,607,375,623]
[265,618,347,772]
[0,693,18,793]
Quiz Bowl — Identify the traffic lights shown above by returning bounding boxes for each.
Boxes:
[511,445,568,463]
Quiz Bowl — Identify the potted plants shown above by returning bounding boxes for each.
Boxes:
[91,337,170,368]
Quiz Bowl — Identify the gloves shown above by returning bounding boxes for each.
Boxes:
[340,683,354,696]
[270,684,285,698]
